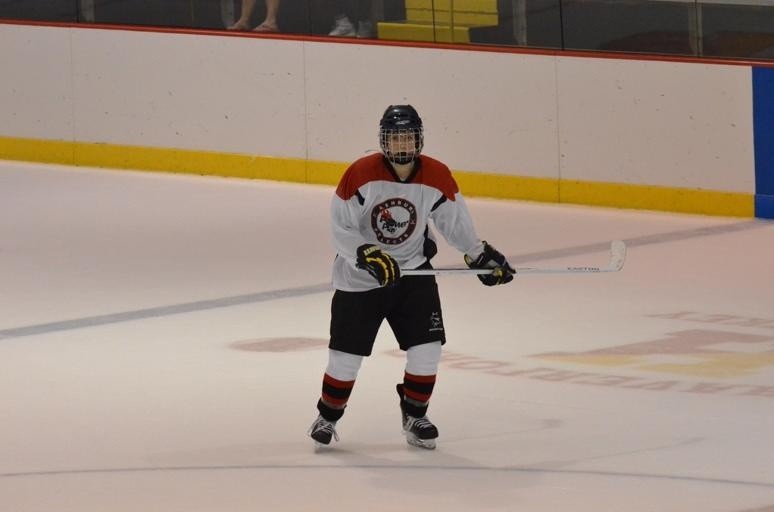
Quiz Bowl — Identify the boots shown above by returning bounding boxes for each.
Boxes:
[311,400,345,445]
[397,383,439,438]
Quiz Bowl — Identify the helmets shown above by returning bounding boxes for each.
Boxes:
[380,105,426,166]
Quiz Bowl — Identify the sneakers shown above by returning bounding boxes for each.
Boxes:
[328,17,378,39]
[230,17,277,33]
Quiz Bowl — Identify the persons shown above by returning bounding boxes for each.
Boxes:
[325,2,386,40]
[225,0,284,33]
[305,105,516,456]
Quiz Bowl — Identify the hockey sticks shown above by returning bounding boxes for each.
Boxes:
[400,239,626,276]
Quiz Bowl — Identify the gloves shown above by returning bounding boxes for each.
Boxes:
[356,242,401,289]
[464,239,516,285]
